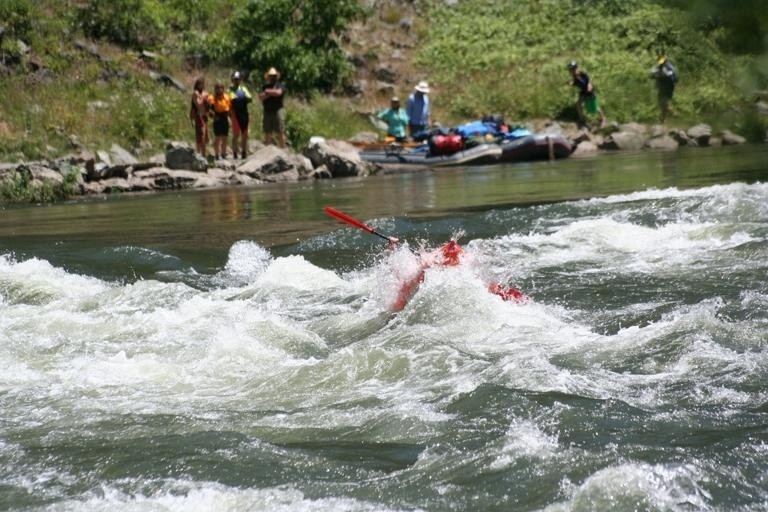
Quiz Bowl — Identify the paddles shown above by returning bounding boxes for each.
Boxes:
[326,207,528,302]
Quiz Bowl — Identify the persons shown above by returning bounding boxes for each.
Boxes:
[388,234,519,309]
[563,61,604,129]
[378,97,411,142]
[406,81,432,133]
[256,67,288,149]
[207,82,231,159]
[226,71,252,158]
[191,77,211,157]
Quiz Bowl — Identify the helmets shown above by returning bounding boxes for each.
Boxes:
[564,62,577,70]
[231,71,241,81]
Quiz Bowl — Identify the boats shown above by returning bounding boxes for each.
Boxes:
[355,122,578,167]
[380,259,526,321]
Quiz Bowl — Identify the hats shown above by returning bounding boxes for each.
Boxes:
[413,81,431,94]
[264,67,280,81]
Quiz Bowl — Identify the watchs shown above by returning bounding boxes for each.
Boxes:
[651,57,678,124]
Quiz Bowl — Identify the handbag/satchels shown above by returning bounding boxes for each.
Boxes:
[430,135,463,155]
